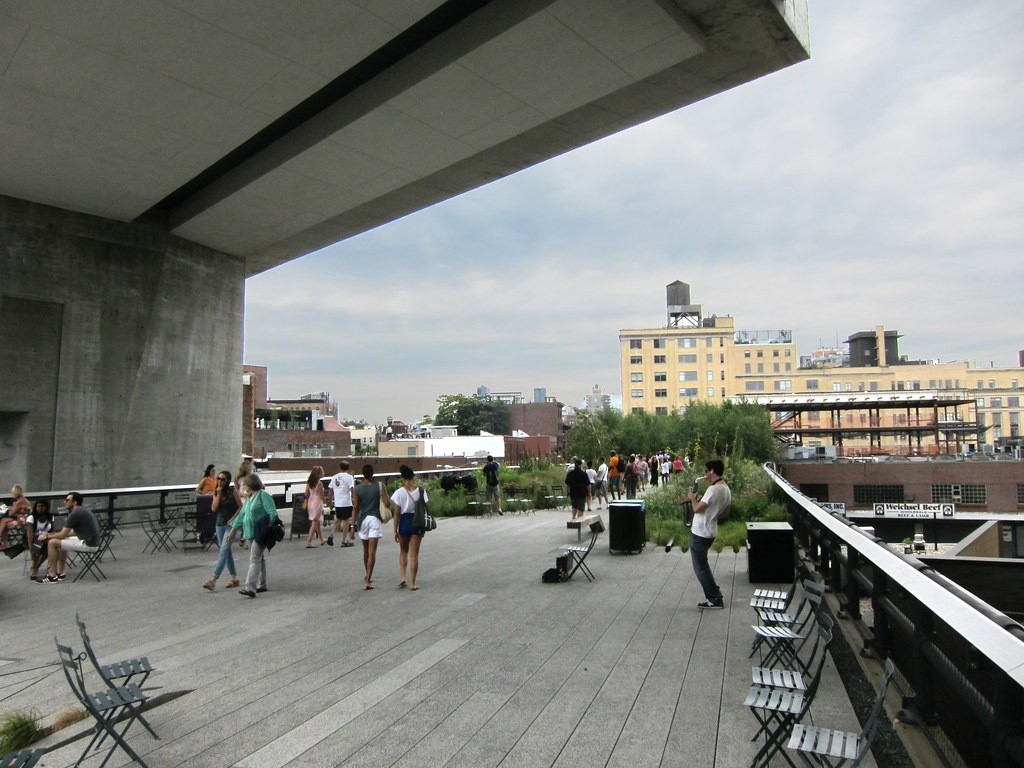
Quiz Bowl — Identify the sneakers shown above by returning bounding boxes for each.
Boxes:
[698,598,723,609]
[57,574,65,580]
[36,573,59,584]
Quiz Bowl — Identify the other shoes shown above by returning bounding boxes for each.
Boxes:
[203,580,215,590]
[340,541,354,547]
[327,535,334,546]
[587,500,612,511]
[225,579,240,588]
[30,575,39,581]
[306,545,317,548]
[256,585,267,593]
[497,510,503,515]
[320,539,327,546]
[239,589,256,598]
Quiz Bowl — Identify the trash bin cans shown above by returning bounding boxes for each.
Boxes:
[609,500,646,554]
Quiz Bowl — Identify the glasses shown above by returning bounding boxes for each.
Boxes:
[705,470,712,473]
[65,498,73,502]
[217,477,228,481]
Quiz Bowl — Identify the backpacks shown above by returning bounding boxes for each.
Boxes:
[616,456,625,473]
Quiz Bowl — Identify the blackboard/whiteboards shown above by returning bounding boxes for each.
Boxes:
[291,493,312,534]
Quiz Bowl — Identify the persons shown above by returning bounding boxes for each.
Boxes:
[350,464,390,589]
[203,470,243,591]
[25,499,55,580]
[328,461,354,547]
[227,474,278,597]
[390,464,428,590]
[687,459,731,608]
[565,450,682,519]
[483,456,503,515]
[195,465,217,494]
[35,492,100,584]
[234,461,253,503]
[305,466,328,548]
[0,486,32,536]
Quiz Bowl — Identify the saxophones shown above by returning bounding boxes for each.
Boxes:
[679,475,708,528]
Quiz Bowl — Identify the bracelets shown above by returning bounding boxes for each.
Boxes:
[350,526,354,527]
[45,535,48,539]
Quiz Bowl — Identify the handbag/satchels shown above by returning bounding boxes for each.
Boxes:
[594,481,602,489]
[412,487,437,531]
[632,464,640,473]
[379,481,393,523]
[302,499,308,510]
[270,518,286,543]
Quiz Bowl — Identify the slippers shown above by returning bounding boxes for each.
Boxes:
[363,575,420,590]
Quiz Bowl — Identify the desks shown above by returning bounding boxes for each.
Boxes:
[609,499,646,554]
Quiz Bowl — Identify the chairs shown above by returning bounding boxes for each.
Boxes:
[138,494,220,555]
[559,521,600,583]
[0,612,156,768]
[0,504,123,583]
[463,485,571,519]
[743,560,895,768]
